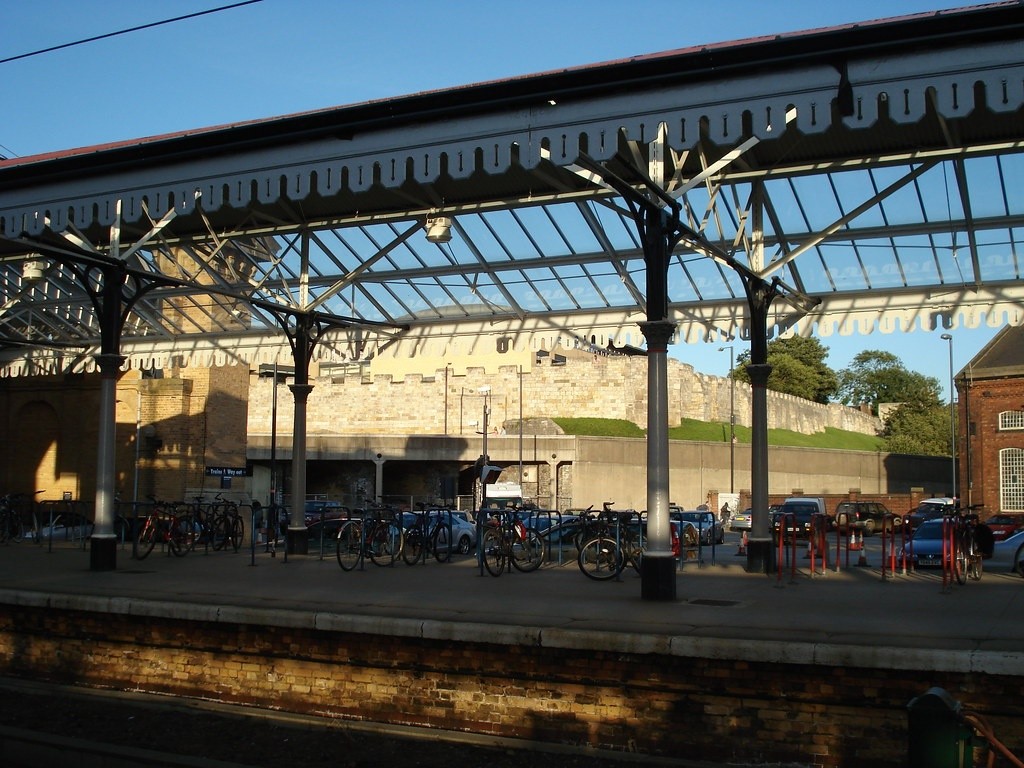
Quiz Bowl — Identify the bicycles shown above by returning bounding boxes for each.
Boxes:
[0,491,648,581]
[937,502,985,586]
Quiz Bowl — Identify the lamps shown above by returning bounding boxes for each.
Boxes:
[425,217,453,245]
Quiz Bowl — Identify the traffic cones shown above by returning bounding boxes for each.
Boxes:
[849,530,864,551]
[735,530,750,556]
[802,536,817,560]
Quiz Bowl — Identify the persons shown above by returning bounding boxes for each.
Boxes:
[965,514,995,580]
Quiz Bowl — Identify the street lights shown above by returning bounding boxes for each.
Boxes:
[718,346,734,494]
[445,361,454,436]
[940,333,956,509]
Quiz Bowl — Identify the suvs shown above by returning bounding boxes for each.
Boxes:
[902,498,955,534]
[832,502,903,538]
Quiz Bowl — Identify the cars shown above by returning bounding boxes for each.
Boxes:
[389,509,477,555]
[730,507,754,531]
[986,511,1024,540]
[671,509,726,547]
[24,510,96,540]
[518,512,585,546]
[116,516,209,547]
[308,519,388,542]
[981,533,1024,576]
[898,519,971,571]
[280,499,350,539]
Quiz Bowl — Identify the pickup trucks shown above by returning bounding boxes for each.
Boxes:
[773,497,826,538]
